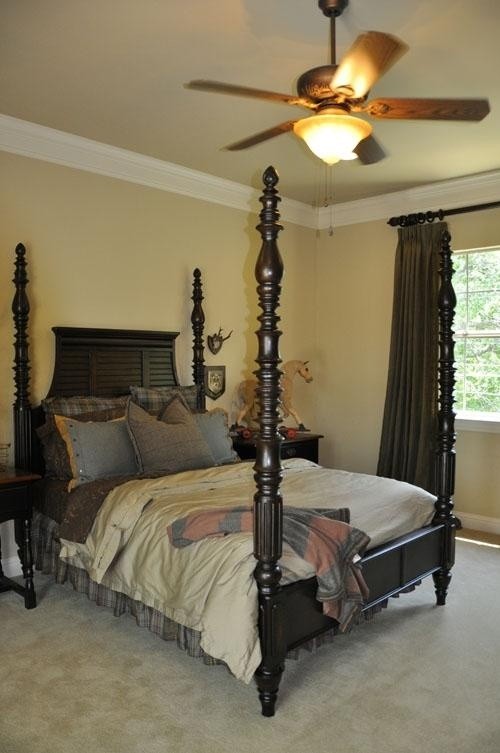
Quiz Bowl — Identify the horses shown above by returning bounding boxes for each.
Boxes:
[235,359,314,431]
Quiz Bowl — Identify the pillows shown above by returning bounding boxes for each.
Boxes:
[40,385,243,493]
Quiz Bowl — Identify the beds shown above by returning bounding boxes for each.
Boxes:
[14,326,462,717]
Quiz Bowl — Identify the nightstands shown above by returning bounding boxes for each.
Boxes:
[1,462,42,610]
[233,433,323,465]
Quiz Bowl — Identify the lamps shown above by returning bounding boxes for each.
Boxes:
[294,104,373,164]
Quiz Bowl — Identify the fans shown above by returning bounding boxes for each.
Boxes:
[183,0,490,165]
